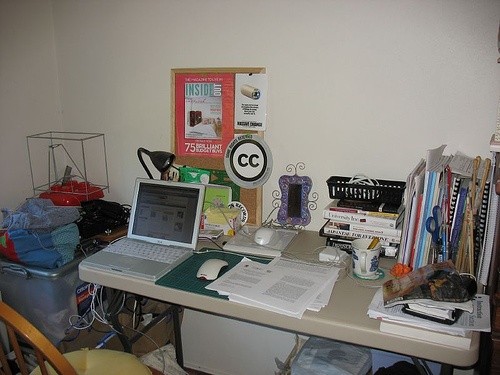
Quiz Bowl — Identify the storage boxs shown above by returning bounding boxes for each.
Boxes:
[0,252,108,348]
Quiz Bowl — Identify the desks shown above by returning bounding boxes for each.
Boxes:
[78,224,480,375]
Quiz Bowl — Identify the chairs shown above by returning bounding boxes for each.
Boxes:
[0,301,154,375]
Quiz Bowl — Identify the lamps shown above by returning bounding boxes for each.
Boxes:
[137,147,176,179]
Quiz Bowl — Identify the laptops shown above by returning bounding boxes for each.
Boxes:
[81,177,206,283]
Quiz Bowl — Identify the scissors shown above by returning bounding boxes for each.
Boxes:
[427,206,442,246]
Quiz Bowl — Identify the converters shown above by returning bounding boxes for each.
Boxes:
[319,246,348,265]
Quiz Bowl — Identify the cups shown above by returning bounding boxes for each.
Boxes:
[351,238,382,276]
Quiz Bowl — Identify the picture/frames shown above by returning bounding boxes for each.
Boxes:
[272,163,319,232]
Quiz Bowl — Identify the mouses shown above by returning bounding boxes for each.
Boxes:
[195,259,228,281]
[254,227,276,245]
